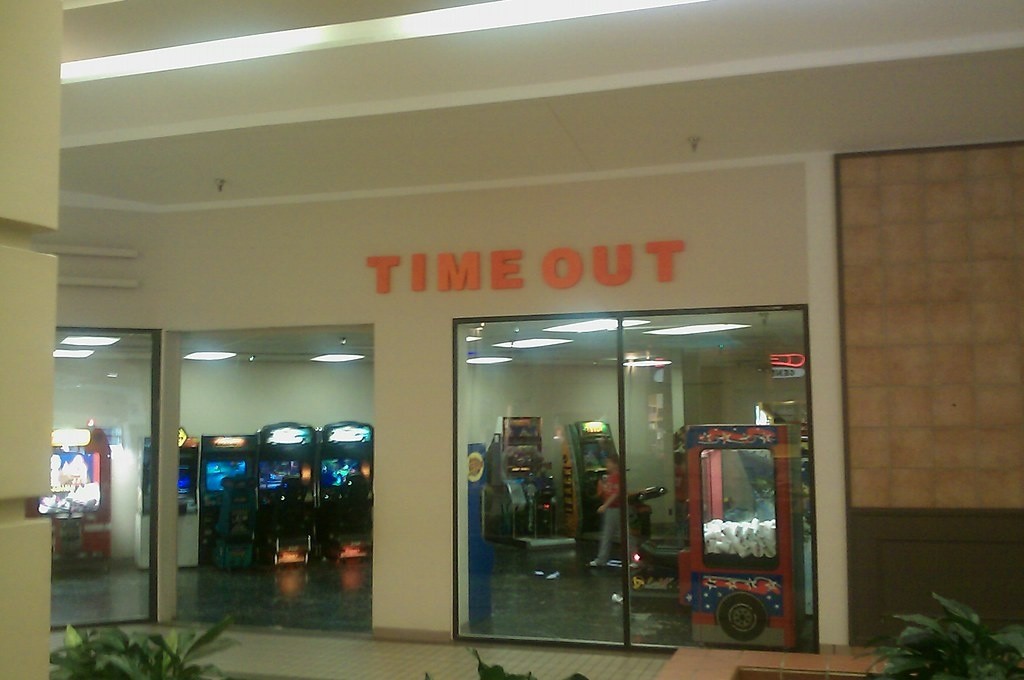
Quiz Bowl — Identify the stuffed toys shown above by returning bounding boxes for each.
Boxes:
[703,517,777,559]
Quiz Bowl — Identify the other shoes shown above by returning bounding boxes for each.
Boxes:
[590,560,606,566]
[630,562,639,568]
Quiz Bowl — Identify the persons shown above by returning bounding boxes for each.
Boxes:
[590,453,639,569]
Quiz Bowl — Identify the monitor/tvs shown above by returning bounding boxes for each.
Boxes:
[509,446,536,477]
[582,441,612,470]
[38,452,101,518]
[176,457,359,496]
